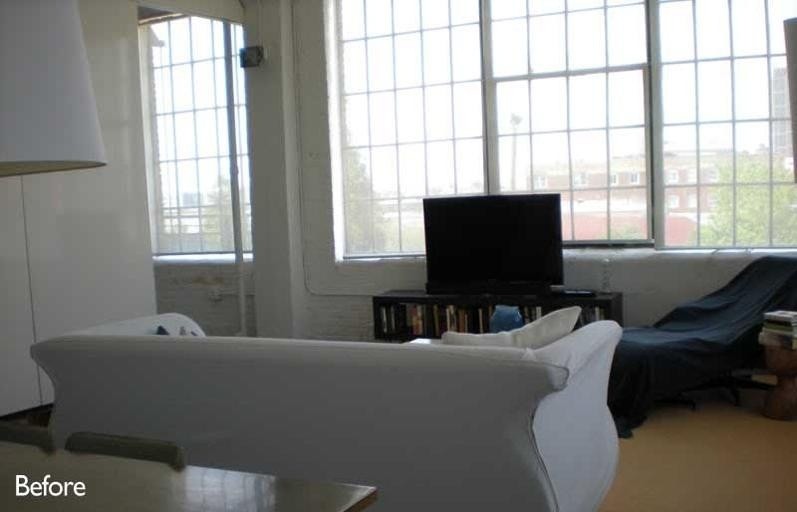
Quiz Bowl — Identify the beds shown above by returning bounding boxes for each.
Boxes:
[763,345,797,422]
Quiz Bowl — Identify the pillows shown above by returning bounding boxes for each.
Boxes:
[440,305,584,350]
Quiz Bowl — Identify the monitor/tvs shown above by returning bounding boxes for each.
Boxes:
[423,194,563,295]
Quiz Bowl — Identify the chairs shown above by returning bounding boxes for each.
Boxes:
[606,255,797,412]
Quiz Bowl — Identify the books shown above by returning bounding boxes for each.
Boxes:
[758,310,797,350]
[377,302,605,338]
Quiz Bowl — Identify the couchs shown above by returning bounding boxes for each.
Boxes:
[29,309,622,512]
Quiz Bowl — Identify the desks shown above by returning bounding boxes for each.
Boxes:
[0,437,378,512]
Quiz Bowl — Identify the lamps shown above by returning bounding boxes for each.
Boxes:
[1,0,110,181]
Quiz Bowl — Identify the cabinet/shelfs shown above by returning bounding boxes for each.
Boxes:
[372,288,622,342]
